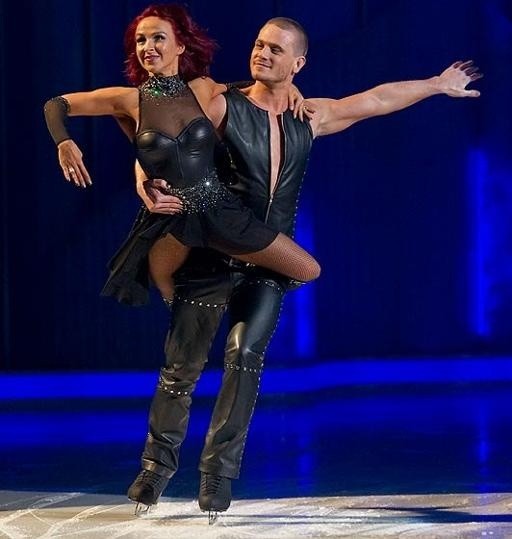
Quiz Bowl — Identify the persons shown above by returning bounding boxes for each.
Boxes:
[43,6,321,312]
[128,17,483,509]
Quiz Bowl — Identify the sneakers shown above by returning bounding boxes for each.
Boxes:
[128,470,170,506]
[199,470,232,512]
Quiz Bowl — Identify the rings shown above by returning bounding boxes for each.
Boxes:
[302,106,309,111]
[69,166,75,173]
